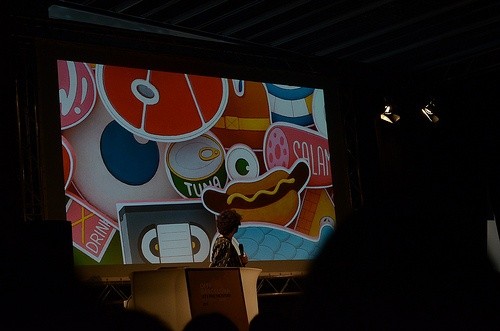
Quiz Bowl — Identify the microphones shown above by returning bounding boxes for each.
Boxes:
[239,244,245,267]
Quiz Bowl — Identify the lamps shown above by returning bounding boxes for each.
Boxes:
[380,106,402,124]
[419,101,441,123]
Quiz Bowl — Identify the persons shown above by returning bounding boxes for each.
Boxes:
[209,209,248,268]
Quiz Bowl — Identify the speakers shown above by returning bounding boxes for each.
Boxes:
[1,221,76,281]
